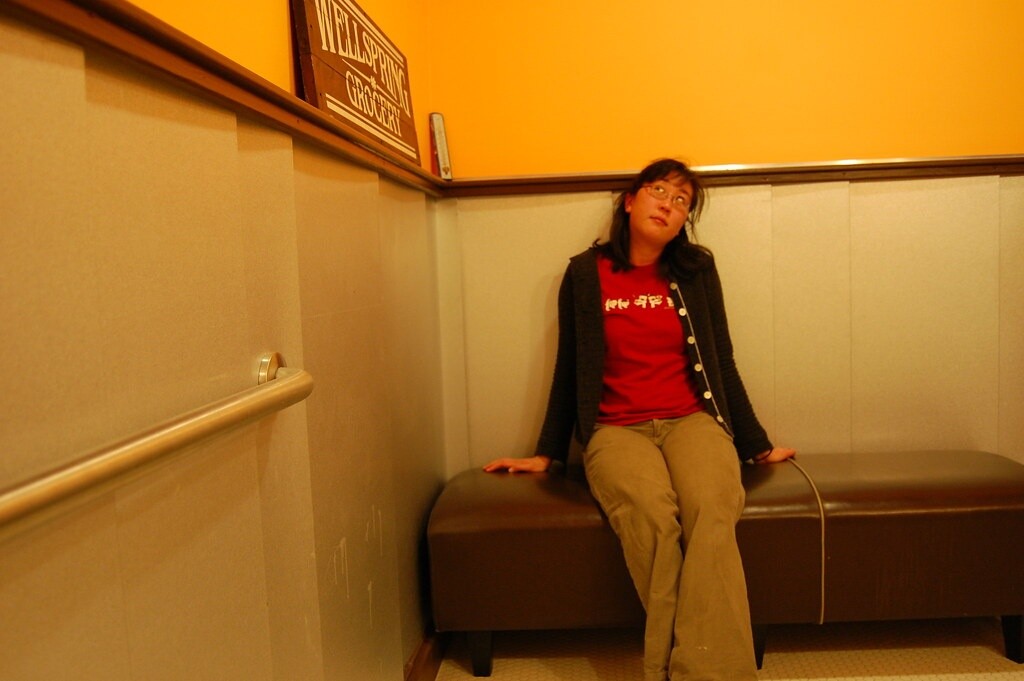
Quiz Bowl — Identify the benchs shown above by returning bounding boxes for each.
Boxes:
[427,448,1024,678]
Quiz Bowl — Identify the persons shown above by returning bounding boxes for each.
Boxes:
[481,159,797,681]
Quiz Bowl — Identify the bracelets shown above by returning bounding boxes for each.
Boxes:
[756,449,772,461]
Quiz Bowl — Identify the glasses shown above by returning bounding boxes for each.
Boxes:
[642,184,691,211]
